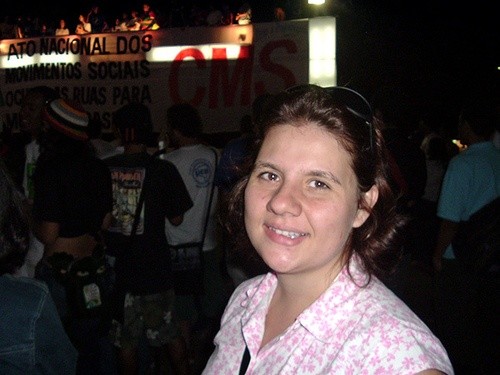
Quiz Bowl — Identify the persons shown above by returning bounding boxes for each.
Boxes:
[18,96,115,375]
[1,169,79,374]
[100,100,194,374]
[218,94,290,323]
[202,83,453,374]
[372,93,427,216]
[157,102,230,369]
[434,96,500,274]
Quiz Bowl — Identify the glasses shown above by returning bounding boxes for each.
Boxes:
[278,83,375,156]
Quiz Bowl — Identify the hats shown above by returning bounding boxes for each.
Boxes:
[43,99,88,135]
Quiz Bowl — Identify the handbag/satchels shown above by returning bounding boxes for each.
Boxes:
[118,255,206,296]
